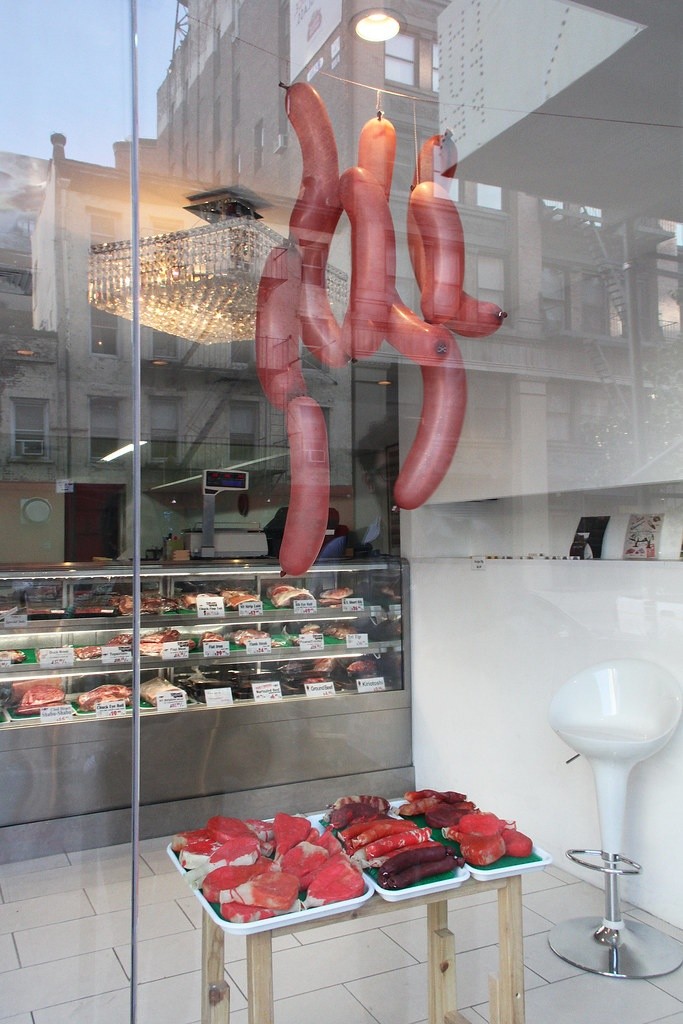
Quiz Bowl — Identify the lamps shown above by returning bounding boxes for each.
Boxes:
[89,201,349,346]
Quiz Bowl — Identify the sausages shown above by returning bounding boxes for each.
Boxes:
[326,789,480,888]
[250,78,507,578]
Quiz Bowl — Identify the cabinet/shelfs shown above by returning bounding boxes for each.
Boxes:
[0,555,416,862]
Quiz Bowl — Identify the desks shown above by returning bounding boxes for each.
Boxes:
[200,797,526,1024]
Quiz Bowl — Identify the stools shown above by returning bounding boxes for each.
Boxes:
[548,658,683,977]
